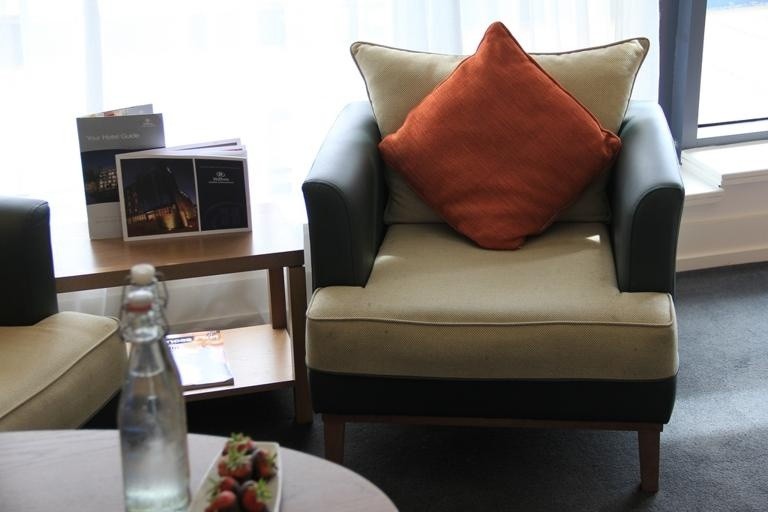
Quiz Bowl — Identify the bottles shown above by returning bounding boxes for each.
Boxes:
[114,264,192,511]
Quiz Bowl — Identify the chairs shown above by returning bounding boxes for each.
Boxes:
[300,98,686,495]
[0,195,128,432]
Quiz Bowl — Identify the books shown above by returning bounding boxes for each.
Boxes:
[166,330,234,391]
[113,136,253,240]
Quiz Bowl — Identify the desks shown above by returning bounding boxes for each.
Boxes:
[49,216,314,427]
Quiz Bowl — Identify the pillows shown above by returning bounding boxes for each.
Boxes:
[349,34,650,226]
[379,19,623,250]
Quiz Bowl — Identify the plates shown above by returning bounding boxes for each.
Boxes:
[186,441,282,512]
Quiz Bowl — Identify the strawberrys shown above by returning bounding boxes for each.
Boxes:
[205,432,278,512]
